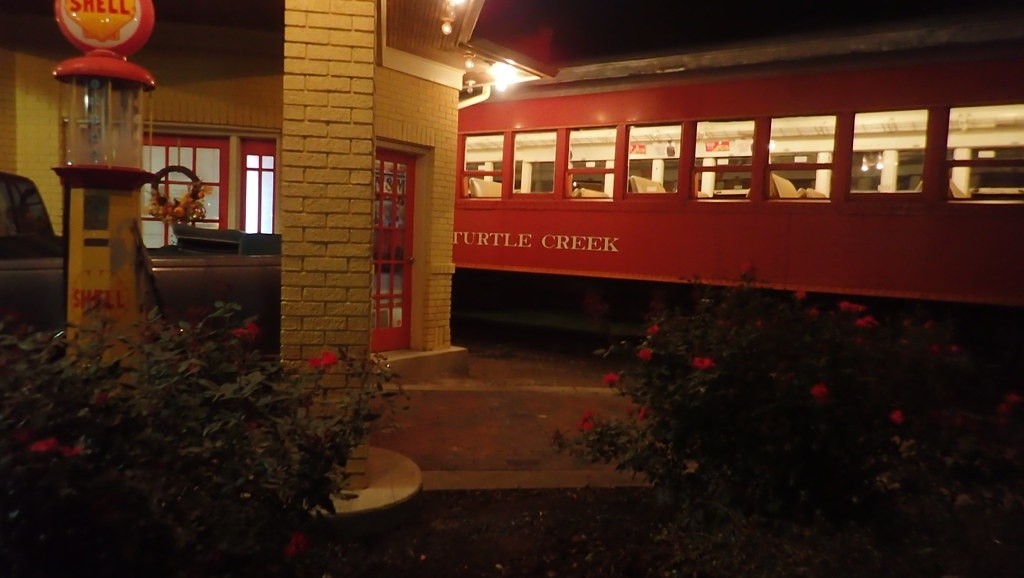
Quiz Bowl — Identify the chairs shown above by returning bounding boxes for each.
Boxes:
[469,177,503,198]
[948,179,971,198]
[630,175,667,193]
[770,174,826,199]
[573,188,609,197]
